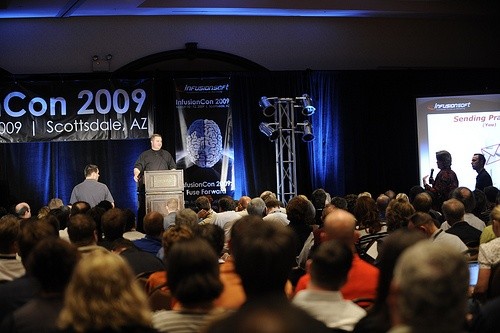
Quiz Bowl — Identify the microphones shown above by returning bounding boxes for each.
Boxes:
[430,169,434,178]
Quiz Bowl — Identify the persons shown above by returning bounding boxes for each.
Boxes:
[471,153,493,190]
[69,164,114,210]
[133,134,177,231]
[422,150,459,204]
[0,188,500,333]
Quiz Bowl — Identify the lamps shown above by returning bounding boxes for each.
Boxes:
[294,120,315,142]
[259,96,279,116]
[294,94,316,116]
[259,121,280,141]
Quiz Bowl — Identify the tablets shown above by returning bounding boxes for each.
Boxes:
[467,261,479,286]
